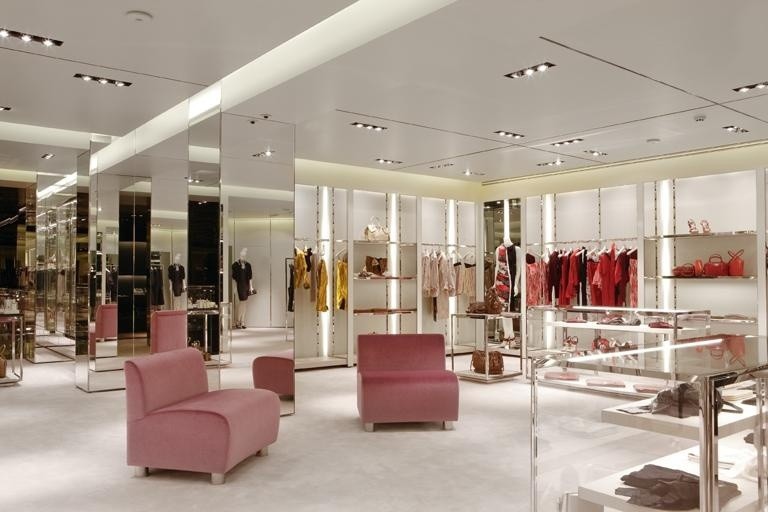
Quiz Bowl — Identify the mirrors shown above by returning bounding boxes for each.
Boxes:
[188,111,296,368]
[72,173,157,393]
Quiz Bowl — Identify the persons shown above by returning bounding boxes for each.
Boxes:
[230,247,257,331]
[166,252,186,309]
[492,233,521,349]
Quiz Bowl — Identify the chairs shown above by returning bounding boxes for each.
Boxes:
[121,344,283,486]
[353,330,463,435]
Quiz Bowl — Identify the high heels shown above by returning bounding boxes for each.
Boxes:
[236,320,246,329]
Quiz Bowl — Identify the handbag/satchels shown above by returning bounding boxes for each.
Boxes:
[727,249,743,276]
[469,288,501,314]
[703,255,727,276]
[650,382,743,418]
[672,260,703,277]
[471,350,502,375]
[365,256,386,275]
[364,216,388,240]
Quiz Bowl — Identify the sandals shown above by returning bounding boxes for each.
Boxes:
[687,218,698,233]
[560,336,578,352]
[700,220,710,233]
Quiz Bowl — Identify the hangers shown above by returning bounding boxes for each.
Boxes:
[528,237,638,263]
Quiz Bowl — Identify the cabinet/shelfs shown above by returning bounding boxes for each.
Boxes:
[452,309,520,386]
[353,239,415,317]
[645,231,755,282]
